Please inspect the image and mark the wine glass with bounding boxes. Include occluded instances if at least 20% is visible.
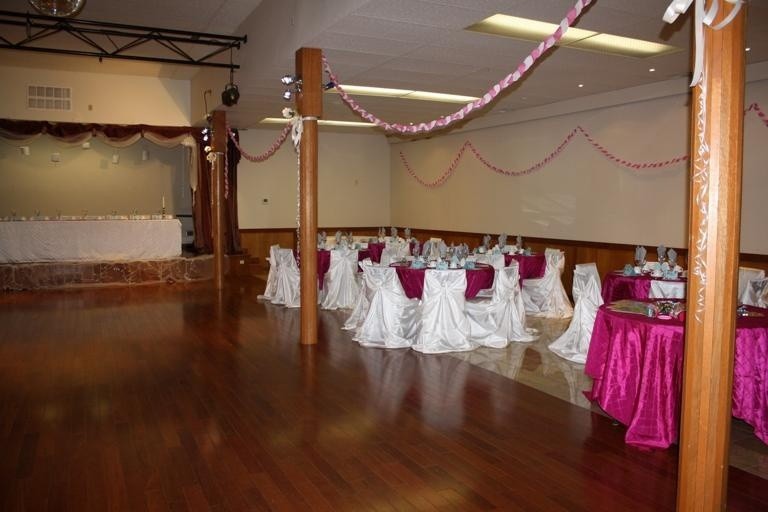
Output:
[413,246,470,268]
[632,255,676,281]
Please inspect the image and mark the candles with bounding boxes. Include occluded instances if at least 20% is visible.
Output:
[162,196,166,208]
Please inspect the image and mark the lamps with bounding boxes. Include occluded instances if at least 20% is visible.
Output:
[201,126,215,144]
[279,71,304,102]
[218,67,242,108]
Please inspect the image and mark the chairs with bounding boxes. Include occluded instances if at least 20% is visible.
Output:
[738,265,767,308]
[545,261,603,364]
[254,225,567,354]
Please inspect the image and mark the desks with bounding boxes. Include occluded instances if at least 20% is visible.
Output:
[0,215,183,264]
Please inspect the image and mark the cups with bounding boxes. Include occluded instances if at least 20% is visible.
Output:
[643,302,686,322]
[479,244,524,254]
